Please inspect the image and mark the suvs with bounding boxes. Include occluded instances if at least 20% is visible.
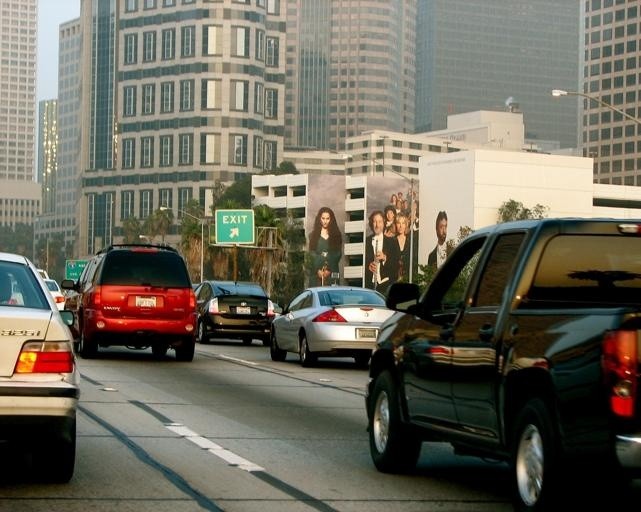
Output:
[61,242,198,362]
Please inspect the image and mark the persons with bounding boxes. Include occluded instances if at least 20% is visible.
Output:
[363,187,419,294]
[308,206,342,286]
[427,211,456,271]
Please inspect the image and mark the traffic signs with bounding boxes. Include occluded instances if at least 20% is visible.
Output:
[65,259,91,281]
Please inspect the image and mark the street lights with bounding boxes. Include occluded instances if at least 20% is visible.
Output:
[337,152,416,282]
[546,86,639,128]
[160,206,207,282]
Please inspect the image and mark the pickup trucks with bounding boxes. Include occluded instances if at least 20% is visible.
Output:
[365,220,640,507]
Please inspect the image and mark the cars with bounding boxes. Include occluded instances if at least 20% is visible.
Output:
[0,252,80,483]
[193,279,277,346]
[268,286,400,368]
